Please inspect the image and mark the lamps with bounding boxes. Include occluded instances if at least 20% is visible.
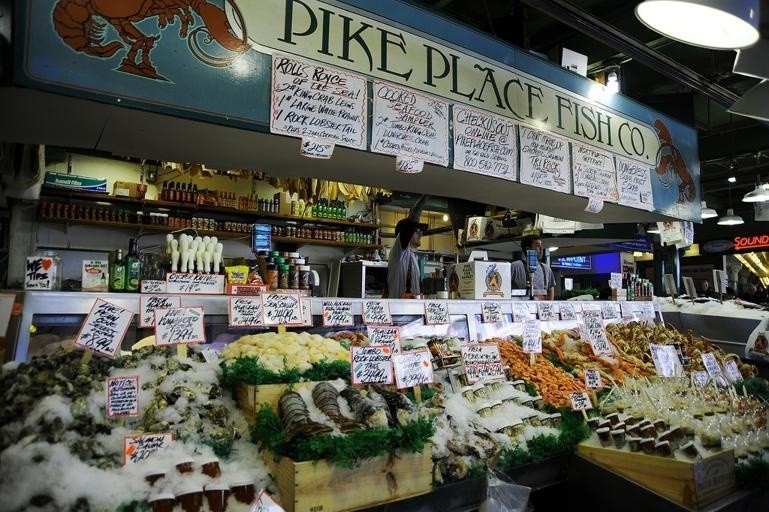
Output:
[603,58,620,94]
[700,23,769,226]
[634,0,761,51]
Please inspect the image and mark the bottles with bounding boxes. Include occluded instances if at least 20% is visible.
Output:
[312,199,346,221]
[162,180,279,212]
[108,235,142,292]
[259,250,311,290]
[272,223,374,244]
[622,273,654,301]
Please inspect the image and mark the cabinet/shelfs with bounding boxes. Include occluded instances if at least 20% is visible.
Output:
[36,145,383,249]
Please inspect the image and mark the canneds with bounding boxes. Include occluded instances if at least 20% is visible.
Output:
[39,199,374,244]
[258,249,311,290]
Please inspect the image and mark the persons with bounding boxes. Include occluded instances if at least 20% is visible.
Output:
[511,234,557,300]
[744,283,769,304]
[386,193,431,299]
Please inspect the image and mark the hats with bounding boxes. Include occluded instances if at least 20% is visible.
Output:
[396,215,429,235]
